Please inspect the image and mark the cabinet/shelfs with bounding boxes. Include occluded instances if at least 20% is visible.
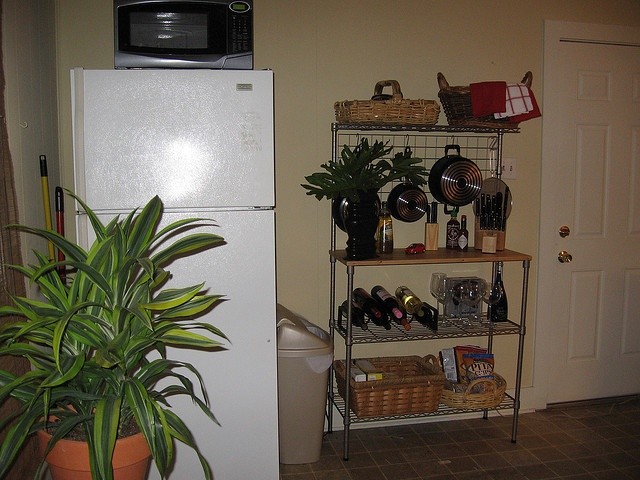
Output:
[323,122,533,461]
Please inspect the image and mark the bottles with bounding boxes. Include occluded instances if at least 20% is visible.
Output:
[447,207,460,250]
[371,285,403,319]
[488,261,508,323]
[378,201,393,254]
[395,285,425,317]
[352,288,381,318]
[415,302,439,330]
[389,296,411,331]
[341,299,368,331]
[371,306,391,330]
[458,216,469,252]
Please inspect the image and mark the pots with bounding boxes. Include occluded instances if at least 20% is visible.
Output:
[429,144,483,215]
[388,146,428,223]
[331,189,381,234]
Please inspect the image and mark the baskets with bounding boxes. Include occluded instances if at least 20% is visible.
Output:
[335,80,440,126]
[436,356,507,409]
[437,72,533,128]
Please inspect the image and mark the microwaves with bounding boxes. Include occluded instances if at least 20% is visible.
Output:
[112,1,255,69]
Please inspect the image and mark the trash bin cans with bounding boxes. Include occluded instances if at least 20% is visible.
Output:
[275,303,333,466]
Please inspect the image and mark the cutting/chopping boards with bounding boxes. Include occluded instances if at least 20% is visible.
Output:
[473,177,513,221]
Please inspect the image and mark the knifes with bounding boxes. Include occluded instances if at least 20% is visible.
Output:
[475,186,509,231]
[425,202,438,223]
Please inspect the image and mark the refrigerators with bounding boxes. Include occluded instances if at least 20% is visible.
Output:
[70,68,280,475]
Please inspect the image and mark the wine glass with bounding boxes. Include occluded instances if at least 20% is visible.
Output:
[452,278,485,331]
[437,278,452,328]
[483,282,502,328]
[429,273,447,317]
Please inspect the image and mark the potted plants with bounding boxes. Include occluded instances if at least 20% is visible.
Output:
[300,137,430,260]
[0,186,234,480]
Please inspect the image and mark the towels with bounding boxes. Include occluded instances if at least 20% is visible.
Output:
[508,88,542,123]
[494,83,534,119]
[469,81,507,117]
